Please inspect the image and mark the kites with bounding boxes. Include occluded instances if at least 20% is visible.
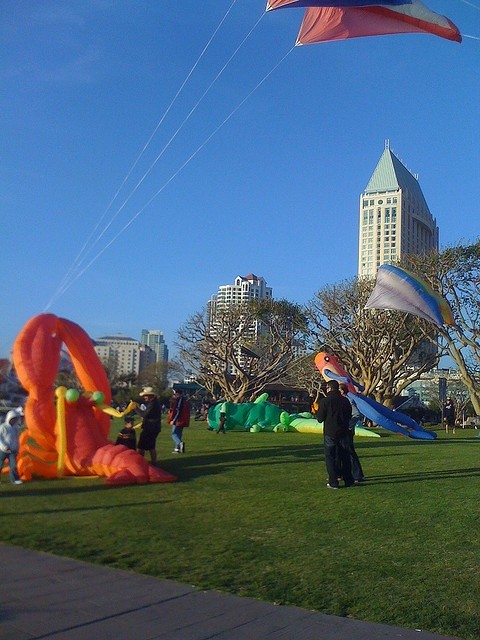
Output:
[262,0,462,49]
[366,262,457,328]
[206,393,382,441]
[314,353,439,442]
[12,314,178,486]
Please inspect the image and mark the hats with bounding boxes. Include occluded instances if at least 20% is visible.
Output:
[124,417,135,423]
[139,386,155,397]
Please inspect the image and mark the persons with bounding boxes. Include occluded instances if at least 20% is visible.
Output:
[167,388,190,453]
[444,396,454,413]
[445,401,456,434]
[337,383,364,486]
[132,387,161,466]
[0,410,23,484]
[315,380,354,488]
[115,417,137,450]
[161,403,165,413]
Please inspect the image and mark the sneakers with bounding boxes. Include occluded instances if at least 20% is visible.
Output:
[327,483,338,490]
[171,448,180,454]
[179,442,185,453]
[11,480,22,485]
[326,476,341,481]
